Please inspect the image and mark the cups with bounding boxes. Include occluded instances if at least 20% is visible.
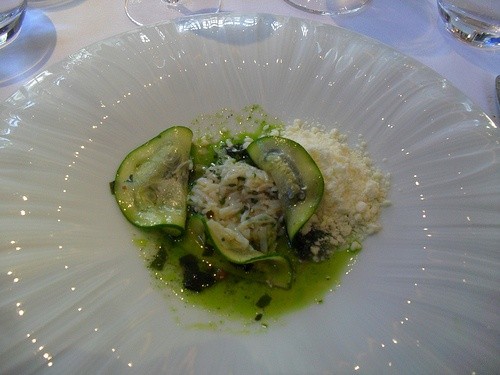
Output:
[436,0,500,49]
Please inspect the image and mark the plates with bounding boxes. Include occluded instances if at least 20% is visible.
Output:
[0,13,500,374]
